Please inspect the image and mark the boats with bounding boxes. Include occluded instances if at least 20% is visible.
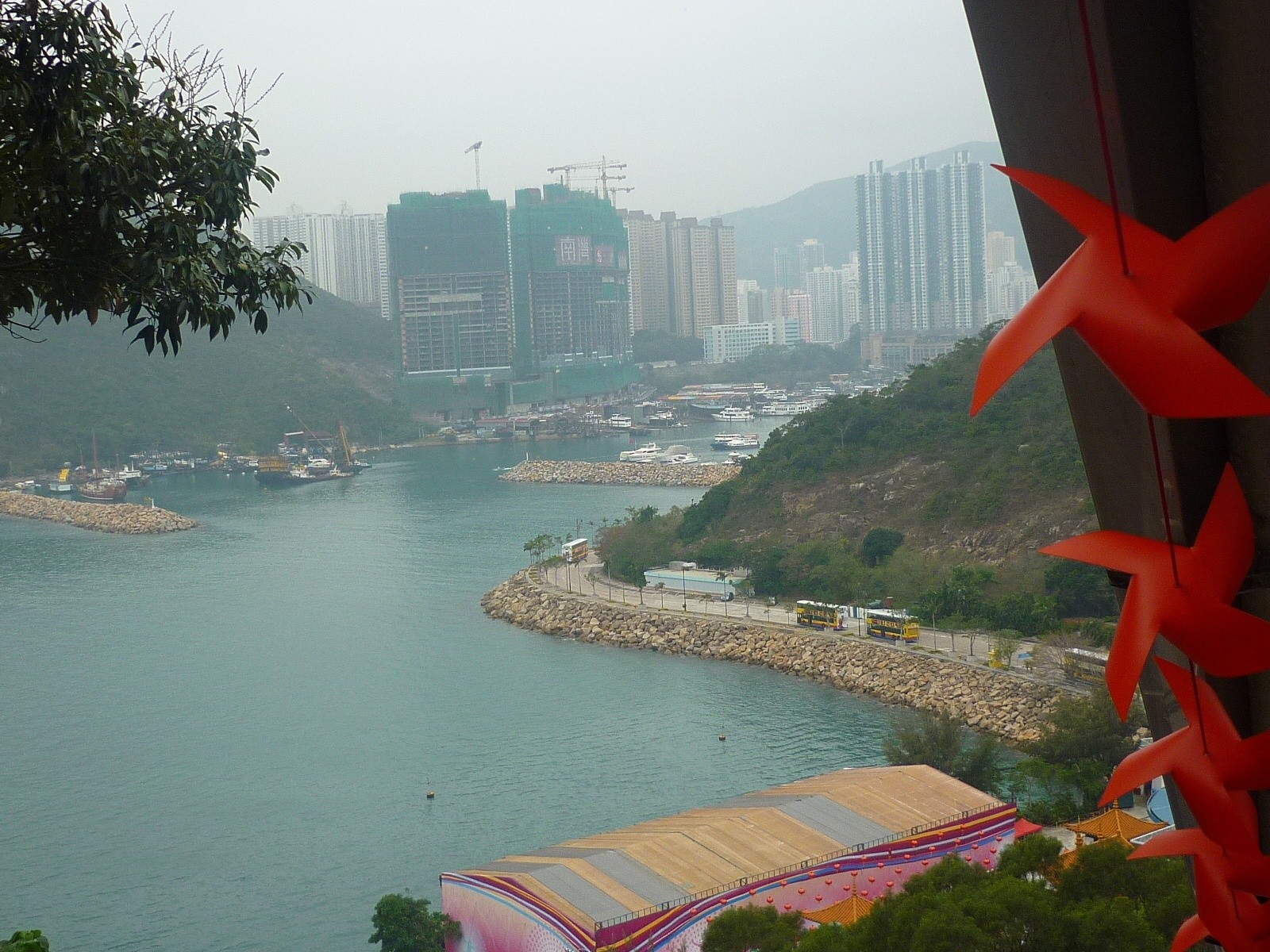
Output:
[710,407,755,422]
[576,411,603,426]
[602,413,632,429]
[756,401,813,416]
[710,432,761,450]
[722,452,753,466]
[15,427,375,505]
[619,441,698,465]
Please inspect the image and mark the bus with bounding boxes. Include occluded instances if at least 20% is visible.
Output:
[1065,649,1140,701]
[866,611,920,643]
[796,600,849,631]
[561,538,589,563]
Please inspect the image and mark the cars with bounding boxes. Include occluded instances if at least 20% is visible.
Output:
[720,592,734,602]
